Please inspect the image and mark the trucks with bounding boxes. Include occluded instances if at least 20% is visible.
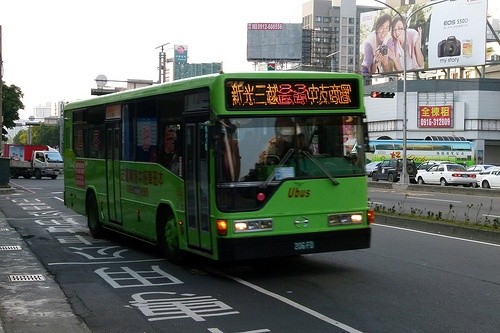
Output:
[10,151,65,180]
[3,144,58,162]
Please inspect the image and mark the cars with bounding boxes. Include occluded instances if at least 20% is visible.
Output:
[363,157,500,191]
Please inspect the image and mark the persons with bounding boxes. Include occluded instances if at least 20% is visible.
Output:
[361,14,395,75]
[387,16,424,71]
[291,133,313,161]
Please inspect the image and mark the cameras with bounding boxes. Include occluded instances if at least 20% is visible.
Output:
[378,45,388,55]
[438,36,461,57]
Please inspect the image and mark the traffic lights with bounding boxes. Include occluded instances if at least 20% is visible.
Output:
[371,90,395,98]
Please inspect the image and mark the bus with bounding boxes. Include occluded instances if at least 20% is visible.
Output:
[63,70,375,266]
